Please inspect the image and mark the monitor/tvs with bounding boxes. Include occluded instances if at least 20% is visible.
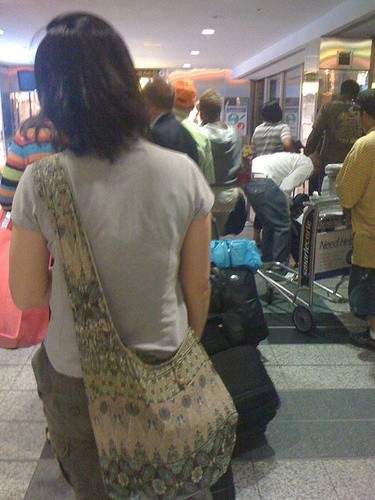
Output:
[17,71,35,91]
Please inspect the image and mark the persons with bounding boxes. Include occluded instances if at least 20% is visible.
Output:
[8,11,214,500]
[335,88,375,348]
[0,80,363,282]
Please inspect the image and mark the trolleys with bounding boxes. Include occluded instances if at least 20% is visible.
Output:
[255,196,351,333]
